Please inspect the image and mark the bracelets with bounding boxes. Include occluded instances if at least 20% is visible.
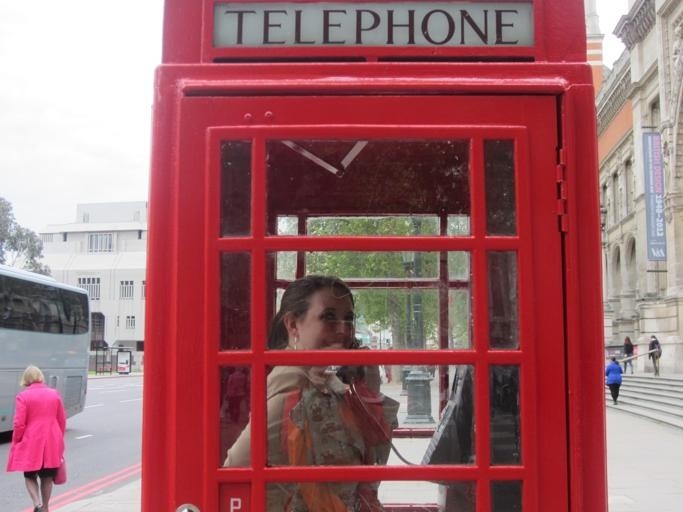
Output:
[359,393,384,404]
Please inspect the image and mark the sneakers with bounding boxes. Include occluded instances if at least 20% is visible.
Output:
[34,505,41,512]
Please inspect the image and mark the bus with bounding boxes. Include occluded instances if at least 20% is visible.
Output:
[0,263,94,433]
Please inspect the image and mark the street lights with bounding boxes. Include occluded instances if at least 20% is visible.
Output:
[396,216,436,429]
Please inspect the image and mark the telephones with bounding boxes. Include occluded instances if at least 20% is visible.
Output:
[335,337,363,385]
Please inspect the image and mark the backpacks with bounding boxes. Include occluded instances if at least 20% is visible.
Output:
[654,344,662,359]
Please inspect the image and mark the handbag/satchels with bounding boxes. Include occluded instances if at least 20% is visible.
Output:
[53,454,67,484]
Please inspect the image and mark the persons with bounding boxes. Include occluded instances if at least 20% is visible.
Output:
[605,356,624,405]
[621,335,635,375]
[226,366,247,426]
[4,364,68,512]
[222,273,401,511]
[647,335,662,375]
